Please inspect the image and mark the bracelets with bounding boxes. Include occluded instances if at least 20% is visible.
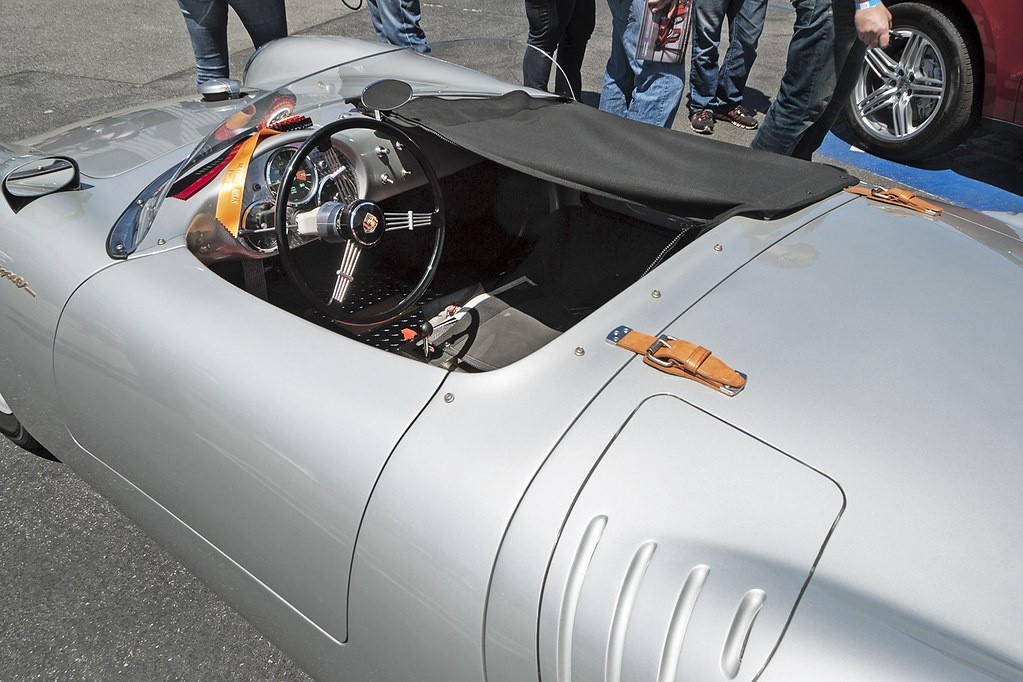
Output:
[854,0,880,10]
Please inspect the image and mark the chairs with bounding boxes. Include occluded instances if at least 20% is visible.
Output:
[391,204,754,372]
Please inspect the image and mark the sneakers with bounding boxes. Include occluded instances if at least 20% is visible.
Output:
[713,105,758,129]
[690,110,713,133]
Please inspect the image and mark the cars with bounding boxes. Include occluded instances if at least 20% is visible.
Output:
[832,0,1022,165]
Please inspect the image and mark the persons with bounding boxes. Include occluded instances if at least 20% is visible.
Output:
[688,0,769,134]
[175,0,290,96]
[599,0,693,131]
[523,0,596,104]
[749,0,893,164]
[365,0,433,57]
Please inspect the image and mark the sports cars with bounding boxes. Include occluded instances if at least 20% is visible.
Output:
[0,32,1023,682]
